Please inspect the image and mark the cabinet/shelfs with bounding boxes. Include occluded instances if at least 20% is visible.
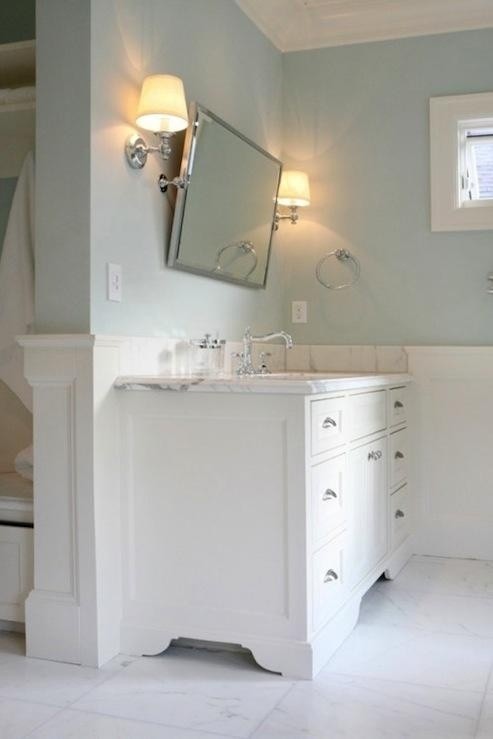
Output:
[113,382,414,680]
[0,496,33,635]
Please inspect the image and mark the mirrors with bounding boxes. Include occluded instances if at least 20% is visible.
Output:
[167,99,284,290]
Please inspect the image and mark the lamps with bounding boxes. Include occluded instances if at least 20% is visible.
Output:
[124,73,190,170]
[273,170,310,231]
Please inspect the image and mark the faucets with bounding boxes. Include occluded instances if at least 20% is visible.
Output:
[243,326,293,375]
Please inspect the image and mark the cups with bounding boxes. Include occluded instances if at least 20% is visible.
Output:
[189,339,226,375]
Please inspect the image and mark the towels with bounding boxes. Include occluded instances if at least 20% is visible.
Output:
[0,148,34,416]
[14,444,33,482]
